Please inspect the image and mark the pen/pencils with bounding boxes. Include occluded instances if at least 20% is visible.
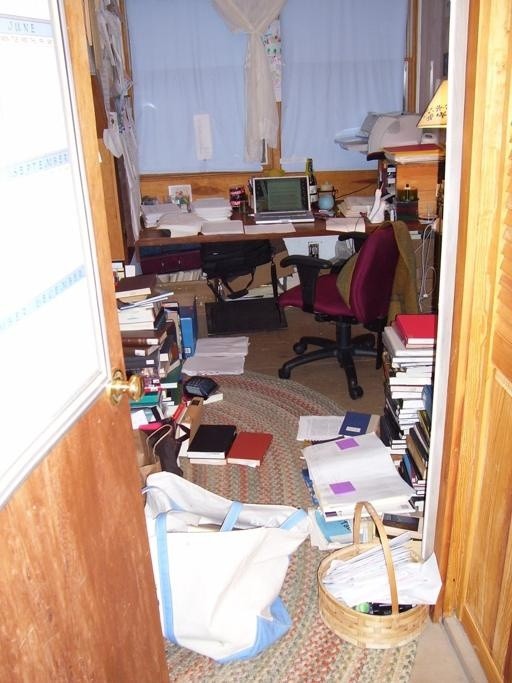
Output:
[247,175,255,195]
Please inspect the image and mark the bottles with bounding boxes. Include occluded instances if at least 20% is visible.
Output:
[318,180,334,211]
[303,157,319,208]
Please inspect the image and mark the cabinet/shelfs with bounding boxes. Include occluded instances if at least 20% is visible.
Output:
[378,159,440,219]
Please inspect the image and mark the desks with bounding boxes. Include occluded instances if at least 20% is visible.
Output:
[135,211,340,260]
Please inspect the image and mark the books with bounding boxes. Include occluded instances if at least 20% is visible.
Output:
[338,143,445,222]
[112,261,274,469]
[296,313,439,551]
[139,197,296,234]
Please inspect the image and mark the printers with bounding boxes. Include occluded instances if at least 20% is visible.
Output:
[334,110,425,153]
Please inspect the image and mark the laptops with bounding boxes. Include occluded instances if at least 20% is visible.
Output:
[253,175,316,224]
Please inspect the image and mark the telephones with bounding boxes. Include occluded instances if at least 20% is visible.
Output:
[366,181,387,224]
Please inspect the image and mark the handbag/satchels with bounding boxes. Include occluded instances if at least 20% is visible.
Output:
[147,418,183,477]
[139,471,314,662]
[201,239,272,279]
[133,431,162,479]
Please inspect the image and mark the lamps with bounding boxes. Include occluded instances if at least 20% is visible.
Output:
[416,79,447,129]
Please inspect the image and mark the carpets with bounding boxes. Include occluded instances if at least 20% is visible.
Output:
[163,370,417,683]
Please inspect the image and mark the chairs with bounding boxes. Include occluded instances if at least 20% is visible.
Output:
[277,225,399,400]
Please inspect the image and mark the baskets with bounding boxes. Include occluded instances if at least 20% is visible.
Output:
[317,502,431,650]
